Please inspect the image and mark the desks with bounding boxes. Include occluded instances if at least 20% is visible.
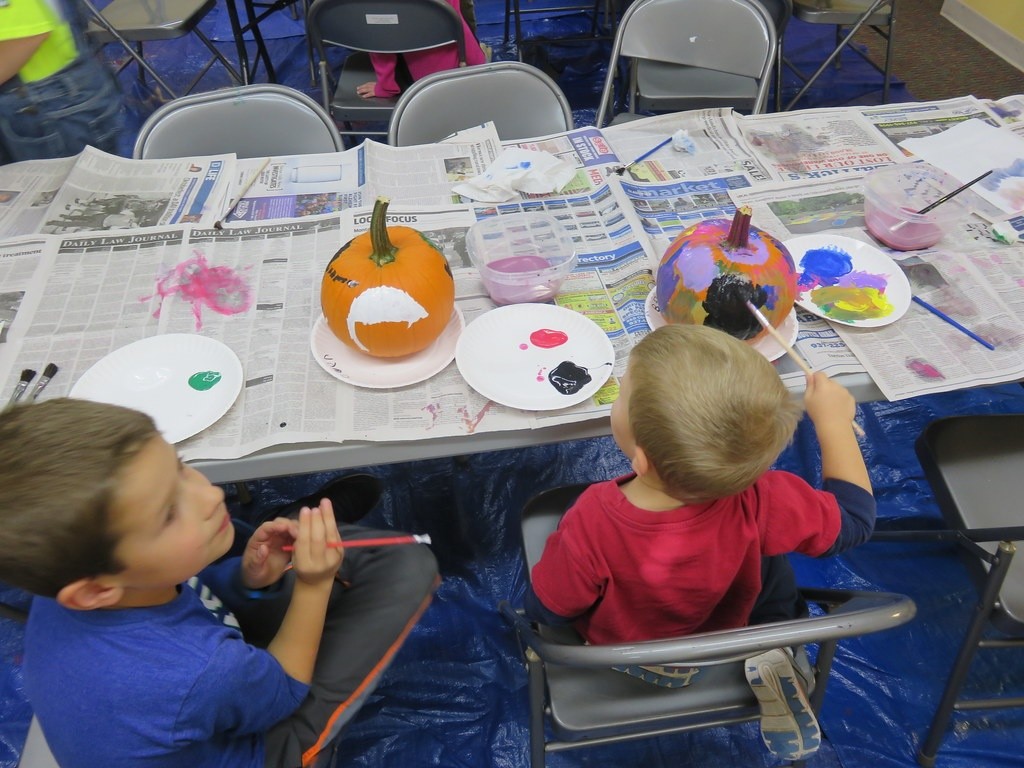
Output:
[1,94,1024,486]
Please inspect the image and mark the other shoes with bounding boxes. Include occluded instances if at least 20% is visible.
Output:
[608,656,704,688]
[745,649,823,760]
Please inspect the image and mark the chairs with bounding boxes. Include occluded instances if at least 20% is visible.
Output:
[498,479,917,768]
[864,414,1024,768]
[80,0,895,160]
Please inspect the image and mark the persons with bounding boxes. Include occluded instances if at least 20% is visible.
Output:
[0,398,443,768]
[0,0,136,160]
[525,324,874,761]
[356,0,493,98]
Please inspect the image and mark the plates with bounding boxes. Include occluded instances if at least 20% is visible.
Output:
[309,302,466,389]
[456,303,615,410]
[781,235,912,327]
[645,285,798,362]
[68,333,243,445]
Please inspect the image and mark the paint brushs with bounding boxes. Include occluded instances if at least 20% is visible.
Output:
[213,158,272,230]
[746,299,866,437]
[282,533,431,552]
[616,137,673,176]
[9,369,36,404]
[911,294,995,350]
[890,169,993,231]
[24,362,58,403]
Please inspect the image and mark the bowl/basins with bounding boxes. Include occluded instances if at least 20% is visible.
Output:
[466,211,578,307]
[864,163,977,250]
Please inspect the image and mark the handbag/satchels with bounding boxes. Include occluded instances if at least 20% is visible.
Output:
[520,29,629,108]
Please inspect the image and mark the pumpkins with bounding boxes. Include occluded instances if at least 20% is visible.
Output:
[656,205,796,346]
[321,195,455,359]
